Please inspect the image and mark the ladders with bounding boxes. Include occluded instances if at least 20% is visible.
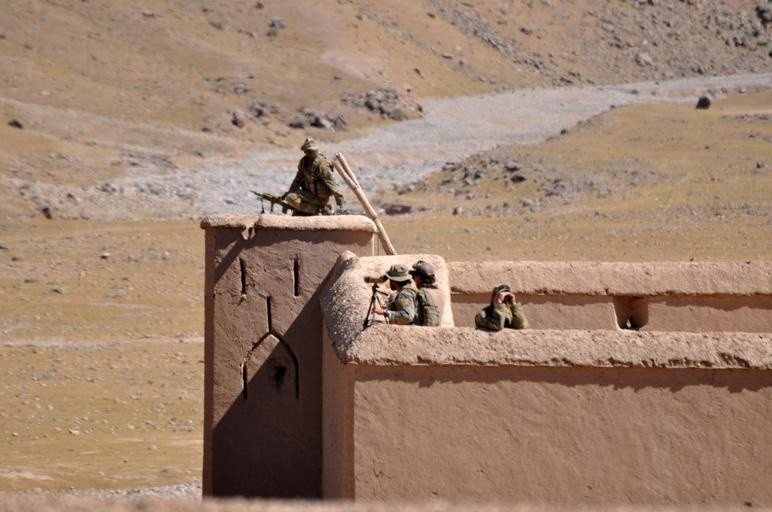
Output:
[334,152,397,255]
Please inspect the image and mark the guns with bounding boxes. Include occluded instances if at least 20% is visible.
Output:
[250,191,312,215]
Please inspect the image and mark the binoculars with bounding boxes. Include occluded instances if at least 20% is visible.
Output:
[505,294,513,300]
[364,282,390,325]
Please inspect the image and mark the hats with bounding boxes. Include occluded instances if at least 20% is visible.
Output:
[408,260,435,280]
[384,262,412,282]
[491,283,511,296]
[301,137,319,152]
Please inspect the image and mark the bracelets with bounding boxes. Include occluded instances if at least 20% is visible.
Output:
[383,309,388,317]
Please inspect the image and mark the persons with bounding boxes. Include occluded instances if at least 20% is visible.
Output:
[372,263,419,325]
[409,260,444,326]
[474,284,529,331]
[286,138,346,214]
